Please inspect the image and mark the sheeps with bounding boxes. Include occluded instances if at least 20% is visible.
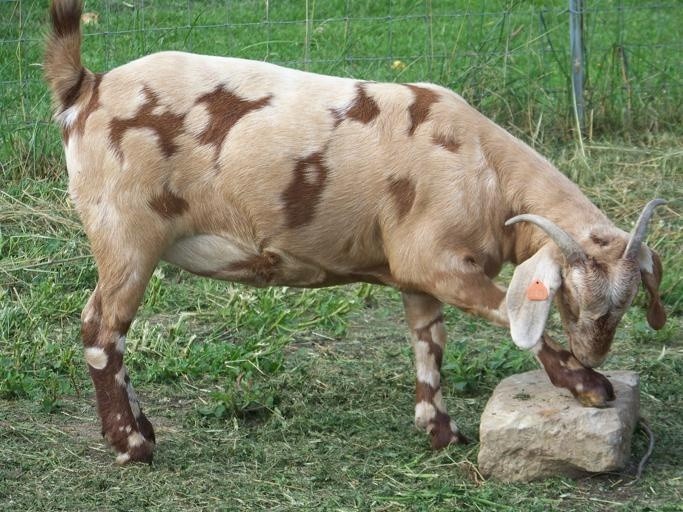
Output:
[41,0,667,468]
